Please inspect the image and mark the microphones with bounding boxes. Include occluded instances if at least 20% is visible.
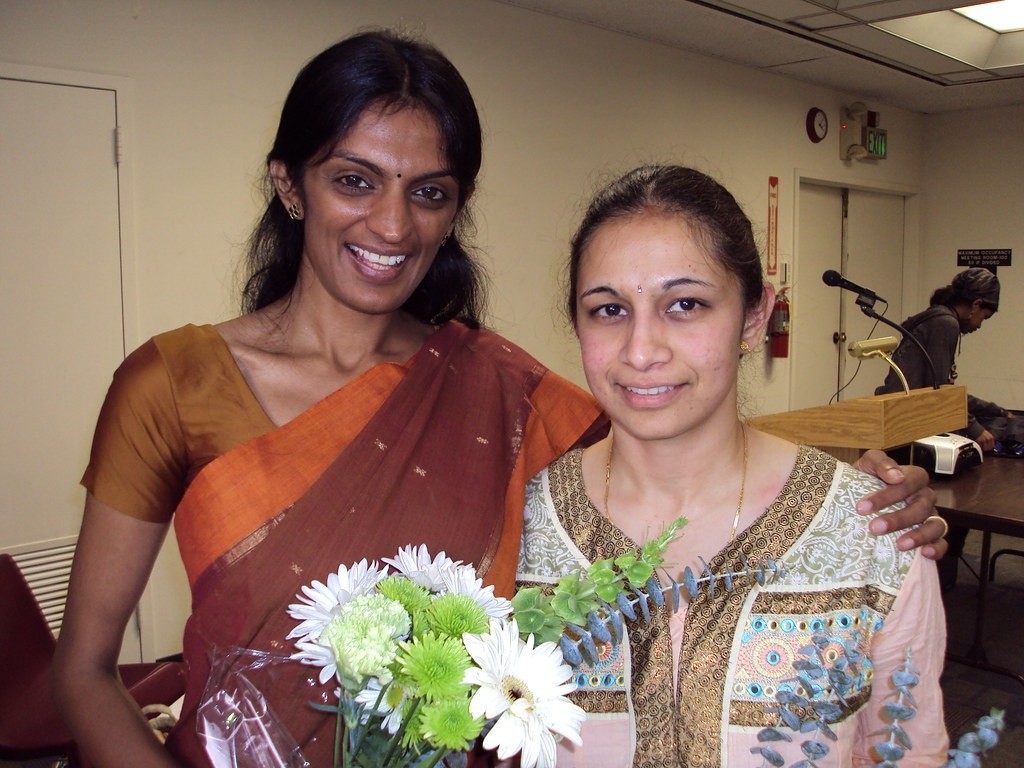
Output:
[822,270,888,306]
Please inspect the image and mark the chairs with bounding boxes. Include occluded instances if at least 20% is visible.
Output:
[0,553,187,768]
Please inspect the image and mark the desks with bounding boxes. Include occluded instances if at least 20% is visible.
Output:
[928,456,1024,687]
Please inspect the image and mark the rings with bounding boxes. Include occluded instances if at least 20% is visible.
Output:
[924,515,948,538]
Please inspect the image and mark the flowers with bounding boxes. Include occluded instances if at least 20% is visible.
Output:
[750,618,1008,768]
[283,517,788,768]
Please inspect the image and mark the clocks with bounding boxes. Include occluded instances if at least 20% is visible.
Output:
[806,107,829,144]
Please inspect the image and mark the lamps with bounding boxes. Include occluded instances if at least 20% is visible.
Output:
[838,101,867,161]
[848,336,911,395]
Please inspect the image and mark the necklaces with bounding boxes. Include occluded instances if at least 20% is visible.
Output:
[604,418,749,545]
[951,305,960,321]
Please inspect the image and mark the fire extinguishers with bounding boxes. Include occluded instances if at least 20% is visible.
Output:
[764,287,790,357]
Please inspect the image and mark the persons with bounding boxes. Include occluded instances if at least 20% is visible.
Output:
[49,24,951,768]
[884,267,1018,596]
[512,164,949,768]
